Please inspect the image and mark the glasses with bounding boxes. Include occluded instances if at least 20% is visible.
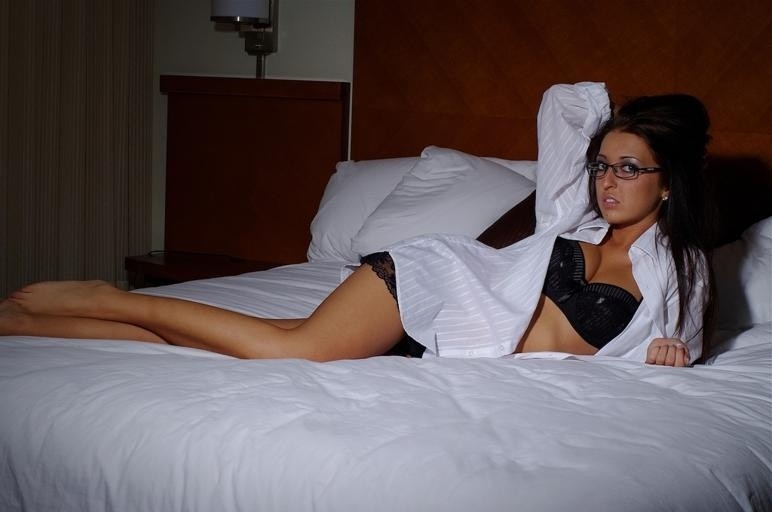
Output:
[586,161,660,179]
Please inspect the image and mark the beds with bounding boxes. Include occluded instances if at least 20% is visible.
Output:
[1,261,771,511]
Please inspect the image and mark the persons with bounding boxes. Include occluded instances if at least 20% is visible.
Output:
[0,80,712,368]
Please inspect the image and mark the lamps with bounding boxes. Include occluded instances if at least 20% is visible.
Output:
[209,1,279,79]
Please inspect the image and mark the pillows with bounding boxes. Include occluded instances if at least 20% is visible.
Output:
[340,148,531,285]
[703,222,768,337]
[477,190,536,248]
[305,157,535,263]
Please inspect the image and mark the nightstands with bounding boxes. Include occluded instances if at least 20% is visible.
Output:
[125,254,285,294]
